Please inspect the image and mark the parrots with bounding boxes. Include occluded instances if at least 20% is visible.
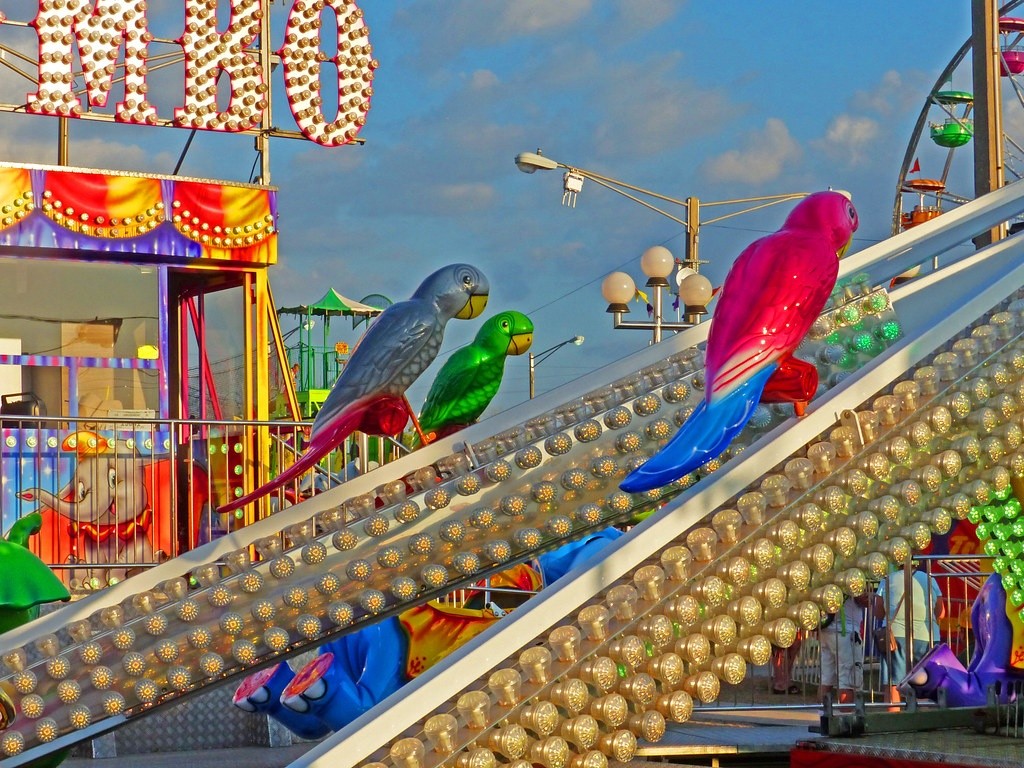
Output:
[616,190,858,493]
[218,264,489,514]
[413,312,534,452]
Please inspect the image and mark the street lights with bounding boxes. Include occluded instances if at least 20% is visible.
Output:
[528,333,586,400]
[512,148,853,289]
[268,317,314,404]
[604,244,711,346]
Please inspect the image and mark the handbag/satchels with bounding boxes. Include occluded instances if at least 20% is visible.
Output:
[873,626,897,653]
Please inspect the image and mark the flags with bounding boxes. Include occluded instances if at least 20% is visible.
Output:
[635,288,653,318]
[672,297,679,310]
[909,158,920,173]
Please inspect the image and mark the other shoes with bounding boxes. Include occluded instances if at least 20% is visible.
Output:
[773,685,801,695]
[817,709,824,716]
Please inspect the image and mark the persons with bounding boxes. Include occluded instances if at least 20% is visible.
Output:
[291,364,299,382]
[772,629,801,693]
[874,564,946,711]
[819,584,870,715]
